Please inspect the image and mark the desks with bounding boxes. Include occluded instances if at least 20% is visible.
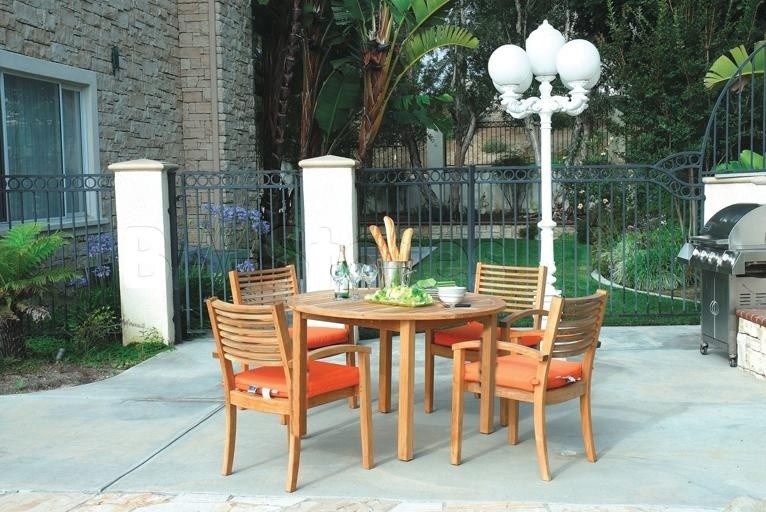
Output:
[285,287,507,461]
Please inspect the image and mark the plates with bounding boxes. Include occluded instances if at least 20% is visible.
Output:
[424,288,439,297]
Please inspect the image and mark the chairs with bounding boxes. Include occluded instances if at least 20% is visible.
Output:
[205,263,371,491]
[425,260,548,427]
[451,288,608,481]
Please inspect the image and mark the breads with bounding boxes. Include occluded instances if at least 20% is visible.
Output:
[399,228,414,261]
[383,216,399,261]
[369,225,392,262]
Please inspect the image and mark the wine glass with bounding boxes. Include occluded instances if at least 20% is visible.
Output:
[359,263,379,299]
[328,262,346,300]
[348,263,361,301]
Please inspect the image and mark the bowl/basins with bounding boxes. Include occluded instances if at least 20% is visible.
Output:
[437,286,468,306]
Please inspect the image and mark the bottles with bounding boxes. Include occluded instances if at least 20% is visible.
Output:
[333,244,349,300]
